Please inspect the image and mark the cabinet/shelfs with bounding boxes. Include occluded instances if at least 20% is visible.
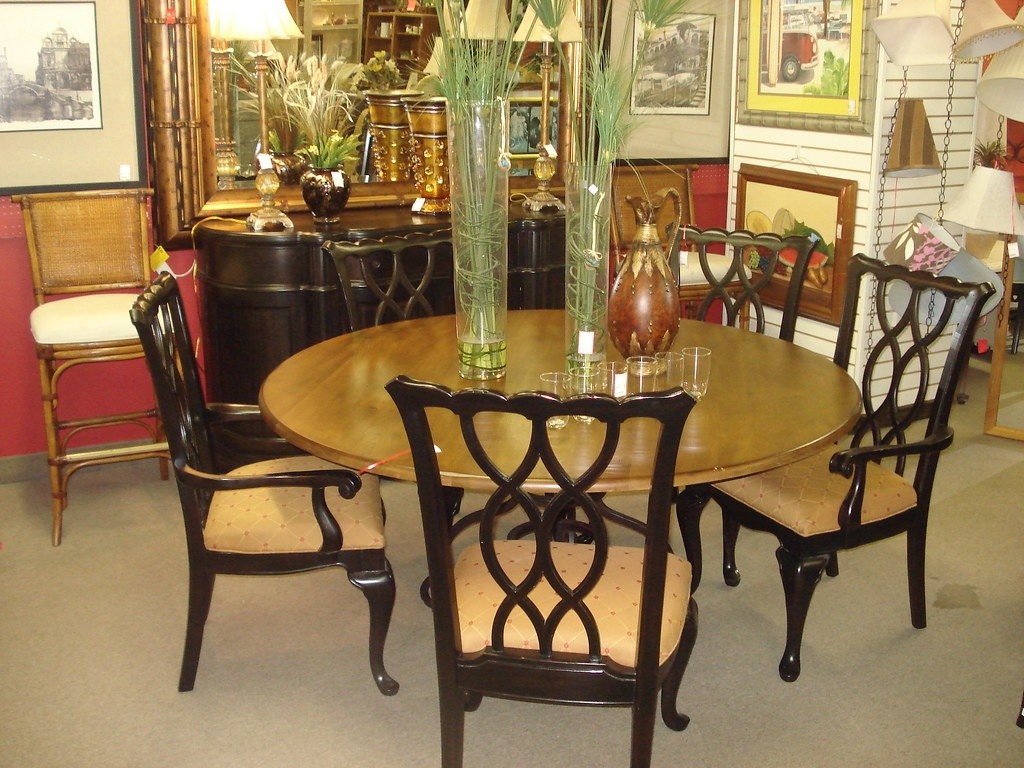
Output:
[271,0,440,82]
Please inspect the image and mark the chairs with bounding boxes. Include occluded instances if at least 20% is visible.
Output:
[128,235,995,768]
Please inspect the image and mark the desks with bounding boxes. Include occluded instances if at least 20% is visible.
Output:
[191,192,565,473]
[257,309,864,598]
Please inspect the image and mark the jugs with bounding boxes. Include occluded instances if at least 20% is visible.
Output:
[607,185,683,374]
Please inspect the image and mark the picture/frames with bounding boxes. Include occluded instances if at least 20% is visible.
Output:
[629,9,716,116]
[734,163,858,327]
[0,2,102,132]
[734,0,882,137]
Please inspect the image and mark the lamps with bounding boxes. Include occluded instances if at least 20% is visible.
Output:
[206,1,291,192]
[455,0,516,55]
[207,0,306,235]
[511,0,587,213]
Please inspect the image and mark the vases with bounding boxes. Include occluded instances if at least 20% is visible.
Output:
[272,152,302,186]
[562,162,615,390]
[214,65,241,177]
[299,164,352,223]
[443,101,512,381]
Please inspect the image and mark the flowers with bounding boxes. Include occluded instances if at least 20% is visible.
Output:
[269,53,362,167]
[225,43,406,152]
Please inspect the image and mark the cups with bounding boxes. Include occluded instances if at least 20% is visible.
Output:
[568,366,601,423]
[539,372,574,430]
[654,351,685,392]
[625,356,658,396]
[597,361,629,400]
[681,347,712,399]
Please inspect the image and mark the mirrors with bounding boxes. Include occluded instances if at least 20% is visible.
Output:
[985,193,1024,443]
[135,0,603,250]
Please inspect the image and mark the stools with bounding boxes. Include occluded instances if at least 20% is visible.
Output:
[611,165,751,332]
[10,188,183,547]
